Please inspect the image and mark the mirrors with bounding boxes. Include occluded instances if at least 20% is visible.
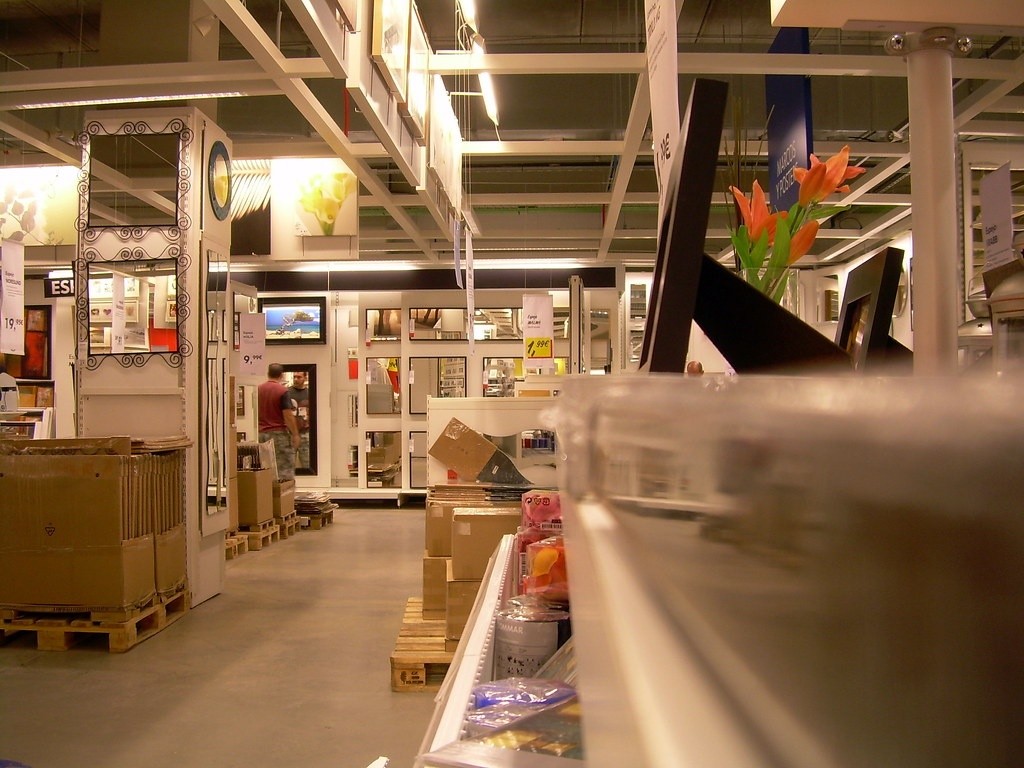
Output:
[74,118,195,244]
[278,361,317,476]
[71,245,193,370]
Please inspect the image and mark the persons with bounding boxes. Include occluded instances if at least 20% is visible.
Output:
[258,363,300,480]
[286,372,310,468]
[687,361,704,377]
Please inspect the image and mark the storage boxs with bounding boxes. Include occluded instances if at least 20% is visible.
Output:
[272,478,297,516]
[419,487,530,650]
[236,467,276,525]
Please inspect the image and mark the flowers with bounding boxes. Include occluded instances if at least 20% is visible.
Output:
[718,82,870,304]
